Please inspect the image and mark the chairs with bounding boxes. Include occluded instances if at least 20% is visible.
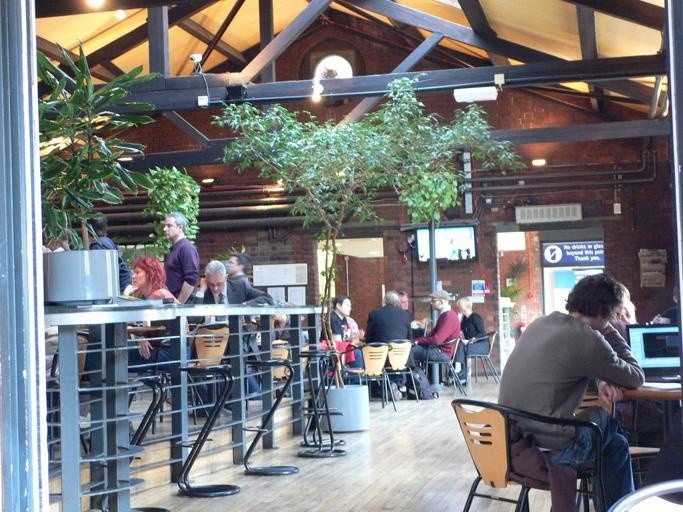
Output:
[42,327,90,459]
[339,329,501,412]
[447,397,609,512]
[155,323,228,425]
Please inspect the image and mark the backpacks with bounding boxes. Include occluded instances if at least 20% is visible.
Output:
[405,366,439,400]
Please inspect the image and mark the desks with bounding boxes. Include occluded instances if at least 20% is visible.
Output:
[579,381,682,494]
[41,297,329,512]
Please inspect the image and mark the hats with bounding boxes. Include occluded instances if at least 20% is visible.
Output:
[427,290,448,300]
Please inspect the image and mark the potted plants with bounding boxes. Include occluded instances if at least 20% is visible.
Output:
[208,71,440,435]
[500,282,521,306]
[34,43,162,307]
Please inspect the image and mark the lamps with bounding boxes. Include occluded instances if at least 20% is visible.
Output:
[491,72,507,94]
[611,186,622,214]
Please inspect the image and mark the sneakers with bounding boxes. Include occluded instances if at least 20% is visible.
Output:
[390,381,403,401]
[452,382,466,386]
[450,371,464,378]
[397,381,407,393]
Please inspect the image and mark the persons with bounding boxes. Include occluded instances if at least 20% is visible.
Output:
[43,210,287,416]
[320,287,489,403]
[614,282,641,345]
[495,274,645,506]
[651,286,680,326]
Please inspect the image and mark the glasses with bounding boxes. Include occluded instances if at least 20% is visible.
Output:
[432,300,440,303]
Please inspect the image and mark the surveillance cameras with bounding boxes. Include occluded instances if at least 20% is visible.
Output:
[189,53,202,62]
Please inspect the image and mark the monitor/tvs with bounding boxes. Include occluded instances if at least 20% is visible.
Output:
[416,225,479,264]
[626,324,683,383]
